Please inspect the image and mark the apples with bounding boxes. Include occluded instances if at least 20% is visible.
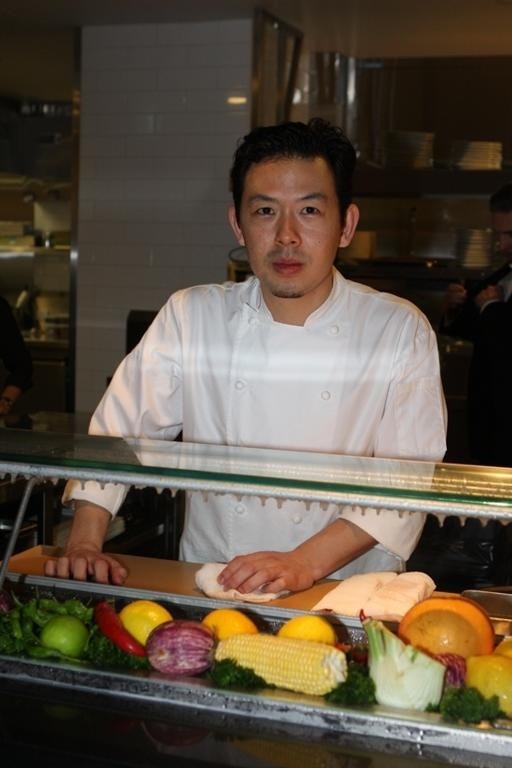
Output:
[39,614,89,657]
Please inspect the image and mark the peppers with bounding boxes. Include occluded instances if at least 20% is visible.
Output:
[0,586,93,649]
[95,599,148,657]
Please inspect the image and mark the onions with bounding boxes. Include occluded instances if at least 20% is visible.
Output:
[145,620,215,676]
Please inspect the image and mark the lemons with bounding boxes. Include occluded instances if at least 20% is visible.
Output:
[466,654,512,719]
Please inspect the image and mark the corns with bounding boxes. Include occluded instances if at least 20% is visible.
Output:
[215,633,348,695]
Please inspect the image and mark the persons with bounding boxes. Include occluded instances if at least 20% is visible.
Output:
[436,180,510,467]
[429,261,483,340]
[0,293,34,428]
[45,117,448,597]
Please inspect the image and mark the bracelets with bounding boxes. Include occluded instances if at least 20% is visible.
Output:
[0,396,14,409]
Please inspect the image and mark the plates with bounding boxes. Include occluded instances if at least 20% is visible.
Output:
[376,128,504,172]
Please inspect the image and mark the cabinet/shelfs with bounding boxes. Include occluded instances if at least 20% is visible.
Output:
[0,429,512,768]
[334,164,512,280]
[0,92,79,411]
